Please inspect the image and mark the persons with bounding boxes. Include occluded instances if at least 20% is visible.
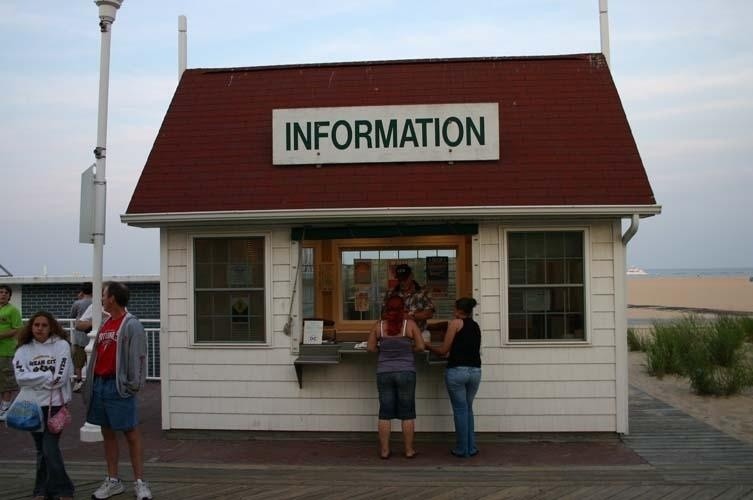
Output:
[77,288,83,299]
[0,284,23,422]
[76,301,109,334]
[366,296,425,459]
[69,281,93,391]
[11,309,78,500]
[423,296,483,457]
[80,281,153,500]
[391,263,436,332]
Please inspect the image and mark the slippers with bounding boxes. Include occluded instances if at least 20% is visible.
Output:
[378,449,419,458]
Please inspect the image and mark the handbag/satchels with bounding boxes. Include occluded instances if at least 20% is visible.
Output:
[46,405,71,435]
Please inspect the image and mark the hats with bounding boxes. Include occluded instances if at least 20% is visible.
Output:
[453,296,476,313]
[394,264,411,279]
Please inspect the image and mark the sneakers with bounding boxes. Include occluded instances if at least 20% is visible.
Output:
[72,379,83,393]
[451,448,478,458]
[0,399,10,410]
[133,478,152,500]
[90,477,124,499]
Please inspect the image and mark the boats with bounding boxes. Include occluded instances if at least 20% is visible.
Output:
[624,265,648,276]
